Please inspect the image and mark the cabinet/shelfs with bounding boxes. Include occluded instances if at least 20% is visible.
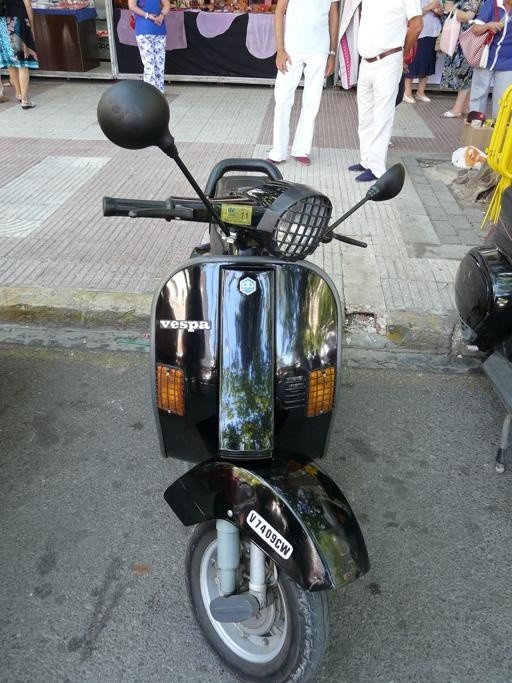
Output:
[28,4,103,73]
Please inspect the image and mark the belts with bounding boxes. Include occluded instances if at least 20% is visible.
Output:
[362,46,404,63]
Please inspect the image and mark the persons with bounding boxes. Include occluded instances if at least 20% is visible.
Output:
[268,0,342,164]
[0,0,38,110]
[437,0,498,118]
[402,0,443,104]
[347,0,425,183]
[463,0,512,127]
[0,78,10,104]
[127,0,171,94]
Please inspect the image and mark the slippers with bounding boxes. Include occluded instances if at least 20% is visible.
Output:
[439,111,461,118]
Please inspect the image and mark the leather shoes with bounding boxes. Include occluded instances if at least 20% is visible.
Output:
[266,156,311,166]
[0,94,36,110]
[347,163,378,182]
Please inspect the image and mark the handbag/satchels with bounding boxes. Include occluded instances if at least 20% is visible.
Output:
[440,19,461,57]
[459,23,495,69]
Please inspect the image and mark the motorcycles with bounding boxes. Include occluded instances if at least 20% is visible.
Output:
[98,80,404,683]
[449,145,512,472]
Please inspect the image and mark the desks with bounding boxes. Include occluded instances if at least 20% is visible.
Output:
[109,5,281,77]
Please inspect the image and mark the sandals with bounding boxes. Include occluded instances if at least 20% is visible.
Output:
[403,92,431,103]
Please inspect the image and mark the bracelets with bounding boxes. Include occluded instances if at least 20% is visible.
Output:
[161,11,166,15]
[328,50,337,56]
[145,12,149,20]
